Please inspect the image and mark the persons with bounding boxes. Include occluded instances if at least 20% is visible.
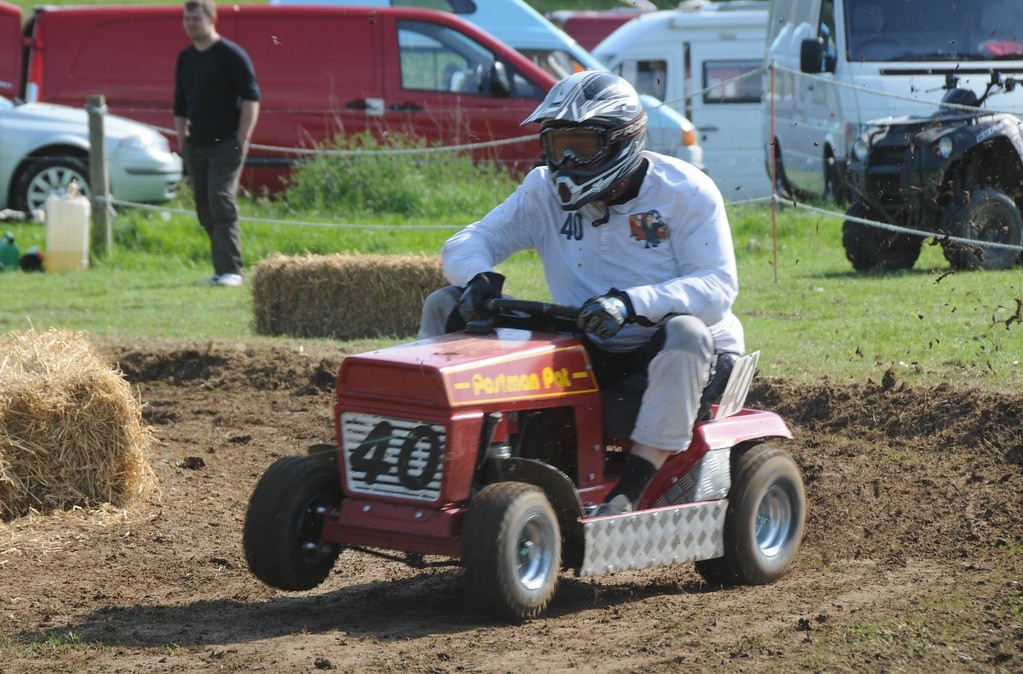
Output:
[172,0,261,286]
[417,71,744,509]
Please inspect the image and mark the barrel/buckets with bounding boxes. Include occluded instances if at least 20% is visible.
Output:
[44,181,90,274]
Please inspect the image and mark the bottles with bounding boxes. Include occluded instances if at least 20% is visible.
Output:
[0,238,19,274]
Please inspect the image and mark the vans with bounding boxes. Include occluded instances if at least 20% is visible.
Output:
[274,0,705,171]
[0,0,564,202]
[761,0,1023,211]
[586,0,770,201]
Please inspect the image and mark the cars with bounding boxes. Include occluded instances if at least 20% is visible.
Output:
[0,93,185,220]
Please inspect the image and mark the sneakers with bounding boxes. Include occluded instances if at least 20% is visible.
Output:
[586,494,632,518]
[216,272,242,287]
[198,275,222,285]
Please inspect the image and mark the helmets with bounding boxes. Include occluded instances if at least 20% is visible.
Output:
[518,70,648,211]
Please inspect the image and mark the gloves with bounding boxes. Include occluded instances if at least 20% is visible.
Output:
[578,288,634,340]
[459,272,505,321]
